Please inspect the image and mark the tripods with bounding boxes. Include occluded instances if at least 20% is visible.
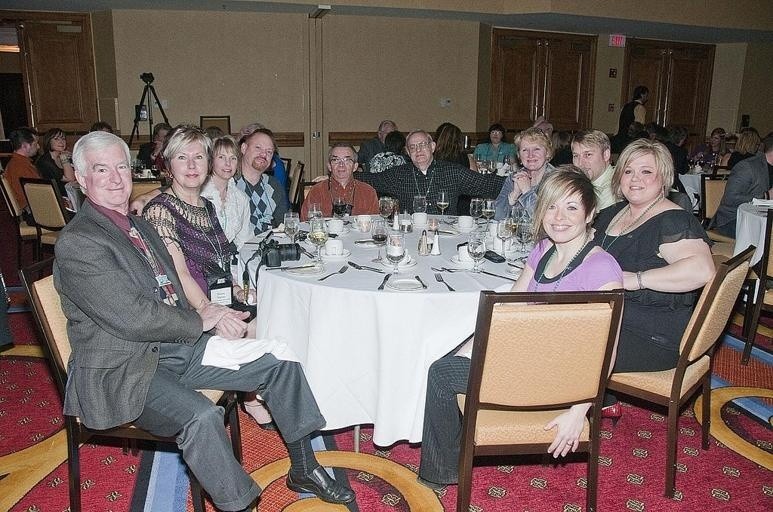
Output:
[127,71,173,149]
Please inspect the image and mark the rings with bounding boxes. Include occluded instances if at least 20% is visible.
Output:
[566,442,573,447]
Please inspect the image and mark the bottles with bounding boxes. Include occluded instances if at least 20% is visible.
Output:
[430,229,440,256]
[419,230,429,255]
[392,210,400,231]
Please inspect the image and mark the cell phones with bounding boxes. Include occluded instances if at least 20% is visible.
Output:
[484,248,506,265]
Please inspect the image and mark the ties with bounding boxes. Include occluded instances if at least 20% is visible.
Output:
[129,224,180,307]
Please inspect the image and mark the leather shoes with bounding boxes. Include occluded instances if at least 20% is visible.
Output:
[286,465,356,505]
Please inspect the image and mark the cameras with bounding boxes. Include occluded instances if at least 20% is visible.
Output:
[253,229,305,270]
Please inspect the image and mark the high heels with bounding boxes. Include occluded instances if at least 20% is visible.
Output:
[240,401,279,431]
[600,403,622,426]
[417,476,448,491]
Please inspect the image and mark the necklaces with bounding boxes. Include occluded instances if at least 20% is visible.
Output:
[172,185,226,273]
[600,195,663,252]
[534,234,588,292]
[412,160,435,206]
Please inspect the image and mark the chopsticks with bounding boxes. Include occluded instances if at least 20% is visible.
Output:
[245,242,260,245]
[480,271,517,282]
[478,221,494,226]
[266,265,315,270]
[505,262,524,270]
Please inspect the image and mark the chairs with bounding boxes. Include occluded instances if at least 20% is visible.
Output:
[709,165,731,180]
[288,162,306,204]
[606,245,756,497]
[740,209,773,365]
[1,175,55,271]
[19,175,71,280]
[457,289,623,512]
[19,255,242,512]
[300,180,318,208]
[700,174,737,244]
[279,158,292,178]
[0,152,15,171]
[712,253,758,337]
[129,177,166,204]
[199,115,231,138]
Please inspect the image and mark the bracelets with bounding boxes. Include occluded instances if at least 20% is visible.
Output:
[636,270,645,290]
[766,190,770,200]
[60,157,70,164]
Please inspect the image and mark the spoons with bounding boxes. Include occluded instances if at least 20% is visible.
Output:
[348,262,385,272]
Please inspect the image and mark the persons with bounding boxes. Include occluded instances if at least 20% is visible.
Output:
[37,127,76,197]
[417,165,625,493]
[5,127,45,226]
[703,127,732,162]
[494,127,558,222]
[240,122,290,212]
[232,130,287,235]
[655,127,672,144]
[534,116,554,139]
[720,127,761,170]
[708,131,773,241]
[300,141,380,222]
[137,122,173,176]
[87,120,114,134]
[30,127,43,167]
[619,85,650,135]
[645,121,659,140]
[473,124,516,165]
[432,122,471,169]
[658,124,692,212]
[202,125,224,141]
[200,134,250,284]
[52,129,356,512]
[571,128,625,215]
[312,129,507,216]
[628,121,644,140]
[140,123,280,432]
[587,139,716,425]
[366,131,412,174]
[357,120,399,172]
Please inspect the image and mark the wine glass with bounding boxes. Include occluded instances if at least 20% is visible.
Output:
[429,217,440,233]
[379,197,394,219]
[285,212,301,244]
[468,235,486,274]
[507,207,519,232]
[151,164,162,184]
[310,219,328,262]
[333,197,348,219]
[413,195,427,212]
[470,198,485,223]
[387,234,405,279]
[372,220,388,263]
[308,203,322,222]
[497,220,512,260]
[483,200,497,232]
[516,223,533,255]
[437,191,449,215]
[489,160,496,173]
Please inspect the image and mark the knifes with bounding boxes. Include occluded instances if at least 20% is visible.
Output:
[413,275,427,289]
[378,273,392,291]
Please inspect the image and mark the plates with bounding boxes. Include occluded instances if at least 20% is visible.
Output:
[380,257,418,268]
[451,255,486,266]
[323,249,351,259]
[286,263,326,276]
[452,224,476,233]
[459,246,473,262]
[385,279,428,290]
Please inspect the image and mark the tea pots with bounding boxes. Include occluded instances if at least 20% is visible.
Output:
[695,165,701,174]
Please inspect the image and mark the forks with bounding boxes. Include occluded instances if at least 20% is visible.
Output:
[319,265,350,281]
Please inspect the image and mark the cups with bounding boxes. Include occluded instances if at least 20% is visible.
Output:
[485,231,502,251]
[398,214,412,234]
[396,248,411,265]
[489,224,498,237]
[355,215,371,234]
[412,213,428,227]
[458,216,474,230]
[506,265,525,278]
[326,220,343,236]
[325,240,344,256]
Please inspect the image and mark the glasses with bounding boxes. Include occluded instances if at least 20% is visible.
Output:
[490,131,502,135]
[409,141,430,152]
[329,157,355,164]
